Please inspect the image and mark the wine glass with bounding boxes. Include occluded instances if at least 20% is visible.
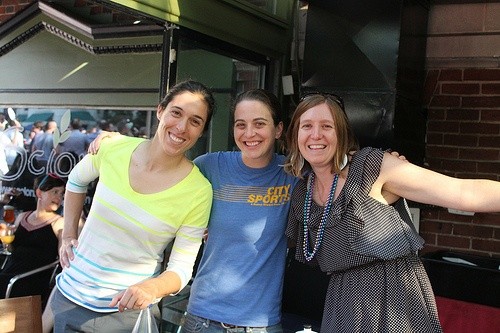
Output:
[0,223,14,255]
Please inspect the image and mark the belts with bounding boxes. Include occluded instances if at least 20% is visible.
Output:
[220,322,235,329]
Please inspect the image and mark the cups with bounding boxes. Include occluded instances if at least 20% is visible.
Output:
[3,206,15,226]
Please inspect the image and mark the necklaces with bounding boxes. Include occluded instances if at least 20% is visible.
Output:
[300,166,339,262]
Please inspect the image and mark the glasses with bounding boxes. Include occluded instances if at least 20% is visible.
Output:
[297,91,345,112]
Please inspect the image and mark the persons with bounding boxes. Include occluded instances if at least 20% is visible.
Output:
[285,91,499,333]
[89,91,408,333]
[0,106,151,216]
[1,173,87,297]
[43,81,214,333]
